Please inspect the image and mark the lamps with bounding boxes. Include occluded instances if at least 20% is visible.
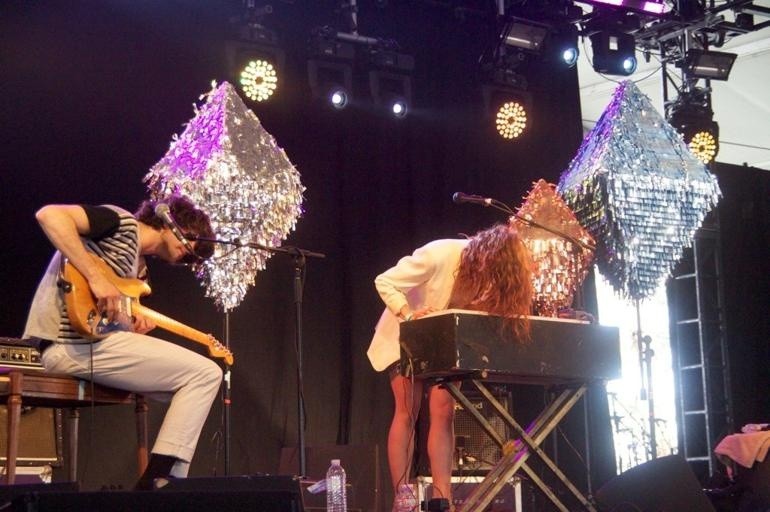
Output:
[229,36,285,113]
[481,85,536,147]
[667,88,721,165]
[546,25,640,77]
[669,45,739,82]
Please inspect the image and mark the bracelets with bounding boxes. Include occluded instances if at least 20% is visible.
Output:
[405,311,415,320]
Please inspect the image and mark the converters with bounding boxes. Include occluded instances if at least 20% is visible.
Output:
[421,498,450,512]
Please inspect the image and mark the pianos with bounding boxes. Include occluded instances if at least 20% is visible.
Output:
[399,308,620,387]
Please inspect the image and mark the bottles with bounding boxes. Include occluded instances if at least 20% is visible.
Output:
[326,459,347,512]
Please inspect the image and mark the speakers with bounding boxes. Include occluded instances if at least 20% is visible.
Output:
[418,391,513,476]
[592,456,716,512]
[155,476,304,512]
[0,365,63,467]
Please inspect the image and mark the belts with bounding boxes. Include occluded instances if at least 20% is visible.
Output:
[39,340,52,354]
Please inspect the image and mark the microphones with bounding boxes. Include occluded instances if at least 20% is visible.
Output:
[452,191,492,206]
[454,436,466,465]
[154,203,193,253]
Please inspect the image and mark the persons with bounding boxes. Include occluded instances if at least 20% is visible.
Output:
[366,222,534,512]
[21,194,226,491]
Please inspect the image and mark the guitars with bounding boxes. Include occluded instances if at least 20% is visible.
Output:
[57,252,235,367]
